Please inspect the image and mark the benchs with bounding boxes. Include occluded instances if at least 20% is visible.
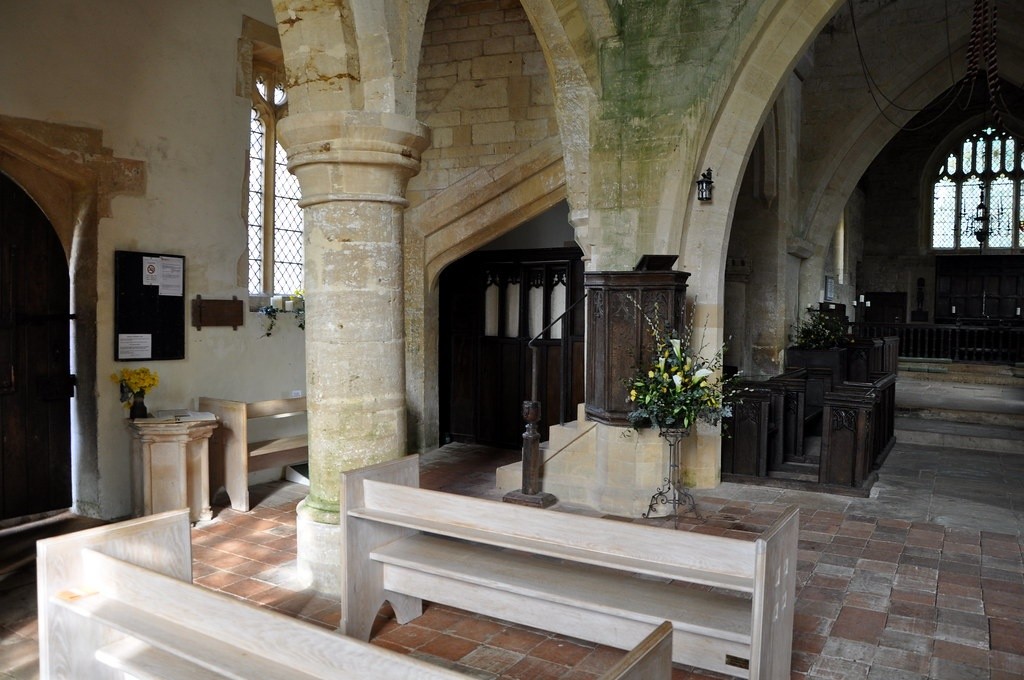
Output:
[34,506,674,679]
[336,453,801,679]
[199,395,308,514]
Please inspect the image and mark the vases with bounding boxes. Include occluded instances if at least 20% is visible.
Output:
[129,390,148,419]
[659,426,688,435]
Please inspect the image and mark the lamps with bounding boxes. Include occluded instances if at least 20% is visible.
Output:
[696,168,714,201]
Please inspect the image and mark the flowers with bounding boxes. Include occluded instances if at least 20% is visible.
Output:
[111,367,158,410]
[789,306,855,351]
[621,298,756,442]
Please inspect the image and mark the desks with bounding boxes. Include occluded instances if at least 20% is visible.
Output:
[124,410,219,526]
